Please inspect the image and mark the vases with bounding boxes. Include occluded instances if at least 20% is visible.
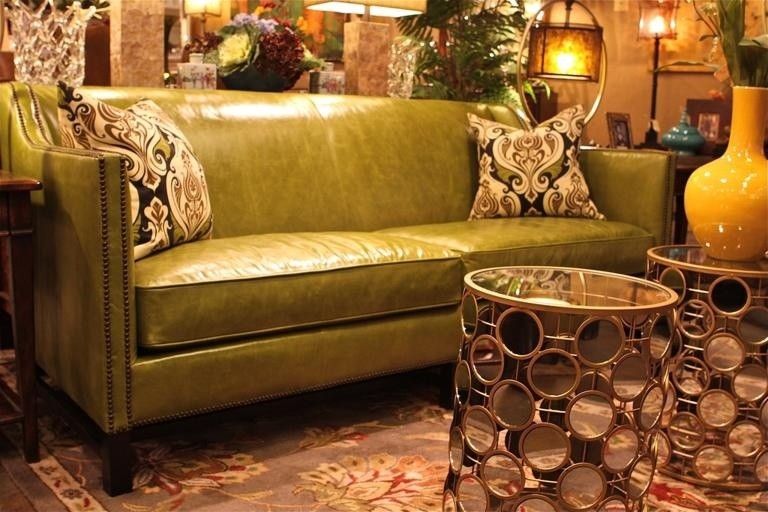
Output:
[683,85,768,261]
[219,61,304,92]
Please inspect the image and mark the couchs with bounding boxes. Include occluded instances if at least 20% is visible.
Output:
[0,80,678,497]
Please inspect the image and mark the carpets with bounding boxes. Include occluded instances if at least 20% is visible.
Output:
[0,335,768,512]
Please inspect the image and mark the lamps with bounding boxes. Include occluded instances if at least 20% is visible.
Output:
[182,0,222,63]
[527,0,604,83]
[634,0,680,151]
[303,0,428,96]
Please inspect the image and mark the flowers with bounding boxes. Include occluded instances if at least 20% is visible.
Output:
[648,0,768,86]
[205,0,327,80]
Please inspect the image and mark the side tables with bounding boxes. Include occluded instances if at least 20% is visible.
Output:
[0,166,44,464]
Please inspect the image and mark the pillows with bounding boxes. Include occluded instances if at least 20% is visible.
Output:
[56,80,214,262]
[465,103,606,223]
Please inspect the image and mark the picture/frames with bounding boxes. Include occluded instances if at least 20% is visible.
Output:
[685,98,733,156]
[606,112,634,150]
